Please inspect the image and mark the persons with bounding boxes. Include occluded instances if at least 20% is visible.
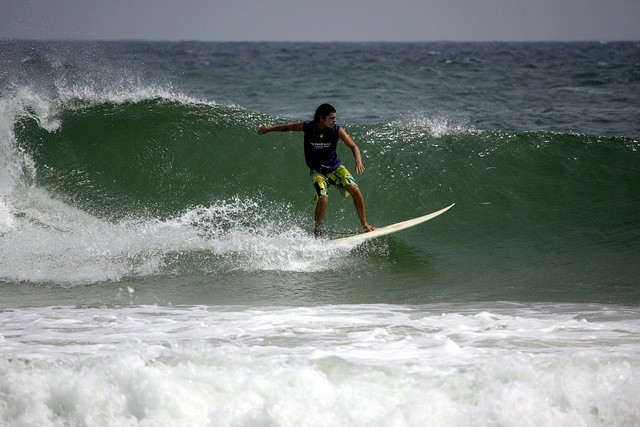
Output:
[257,103,378,238]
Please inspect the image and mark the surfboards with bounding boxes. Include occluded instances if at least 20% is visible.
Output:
[328,202,456,247]
